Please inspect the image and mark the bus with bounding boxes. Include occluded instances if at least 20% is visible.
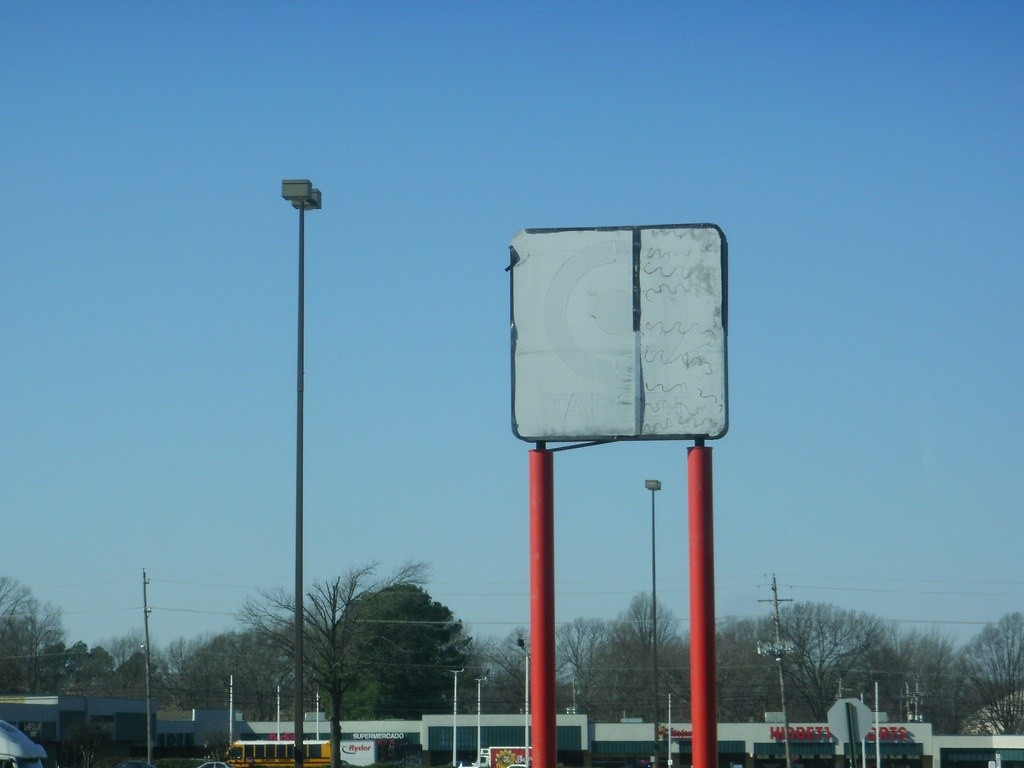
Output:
[222,739,332,768]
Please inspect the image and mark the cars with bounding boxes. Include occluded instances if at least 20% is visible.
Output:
[173,760,230,768]
[114,760,157,767]
[447,761,475,768]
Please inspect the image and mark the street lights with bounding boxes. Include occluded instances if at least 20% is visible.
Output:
[474,676,488,759]
[644,478,662,767]
[449,668,466,768]
[281,179,321,767]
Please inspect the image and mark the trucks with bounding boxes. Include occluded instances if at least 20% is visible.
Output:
[476,747,533,768]
